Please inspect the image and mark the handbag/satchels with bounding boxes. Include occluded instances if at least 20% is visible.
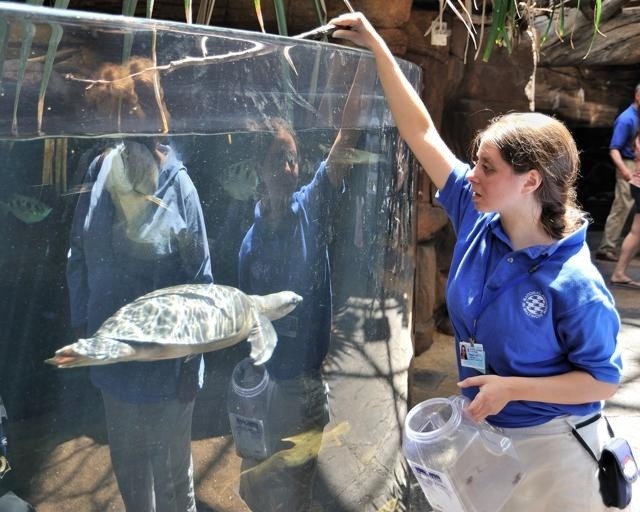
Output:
[597,434,638,509]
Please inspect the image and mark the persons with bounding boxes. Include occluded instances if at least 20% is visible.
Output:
[611,105,640,289]
[66,57,215,512]
[330,9,623,512]
[238,51,375,511]
[596,84,640,263]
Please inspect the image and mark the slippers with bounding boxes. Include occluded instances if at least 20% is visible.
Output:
[610,276,640,289]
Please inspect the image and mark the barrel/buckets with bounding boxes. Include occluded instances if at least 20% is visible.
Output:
[226,356,288,462]
[402,393,527,512]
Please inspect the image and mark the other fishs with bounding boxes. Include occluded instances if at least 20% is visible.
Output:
[212,157,259,201]
[329,144,387,166]
[0,191,51,226]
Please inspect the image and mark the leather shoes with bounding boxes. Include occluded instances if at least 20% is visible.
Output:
[595,251,618,261]
